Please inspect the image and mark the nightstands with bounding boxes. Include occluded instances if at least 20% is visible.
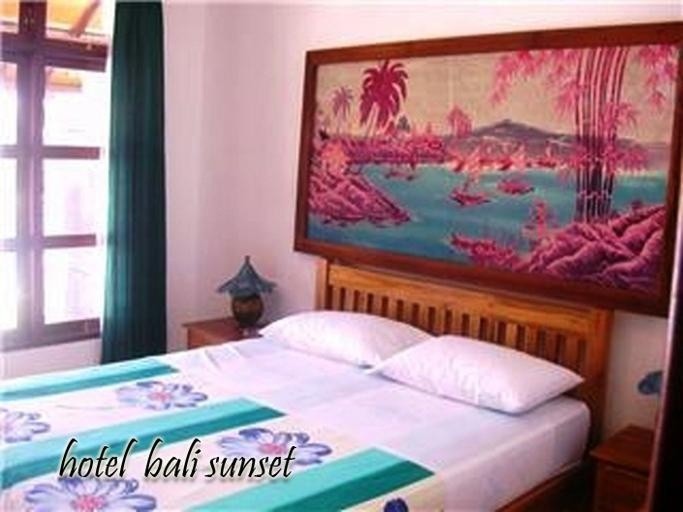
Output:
[588,423,656,512]
[181,315,269,351]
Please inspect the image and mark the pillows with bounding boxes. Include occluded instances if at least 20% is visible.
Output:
[363,334,585,416]
[257,310,437,368]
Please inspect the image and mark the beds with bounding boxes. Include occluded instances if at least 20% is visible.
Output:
[1,256,612,511]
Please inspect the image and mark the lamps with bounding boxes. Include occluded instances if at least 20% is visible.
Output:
[638,370,663,433]
[214,256,278,338]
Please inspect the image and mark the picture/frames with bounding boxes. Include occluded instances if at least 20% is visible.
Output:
[293,19,683,319]
